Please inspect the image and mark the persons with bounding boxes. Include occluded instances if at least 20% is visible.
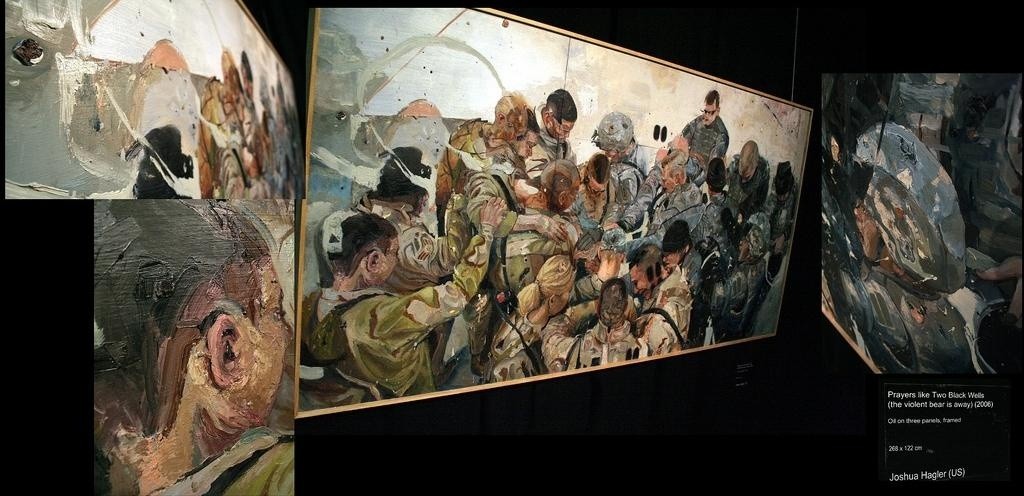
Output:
[181,47,302,197]
[299,83,797,395]
[94,198,296,496]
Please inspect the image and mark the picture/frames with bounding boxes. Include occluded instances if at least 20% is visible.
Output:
[295,7,815,428]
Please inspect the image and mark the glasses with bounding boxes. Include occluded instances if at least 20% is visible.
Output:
[591,184,606,193]
[574,192,580,200]
[704,109,717,116]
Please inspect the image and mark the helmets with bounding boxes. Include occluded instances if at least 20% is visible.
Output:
[597,111,633,151]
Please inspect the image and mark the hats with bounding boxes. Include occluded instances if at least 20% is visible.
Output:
[662,219,690,253]
[379,147,432,192]
